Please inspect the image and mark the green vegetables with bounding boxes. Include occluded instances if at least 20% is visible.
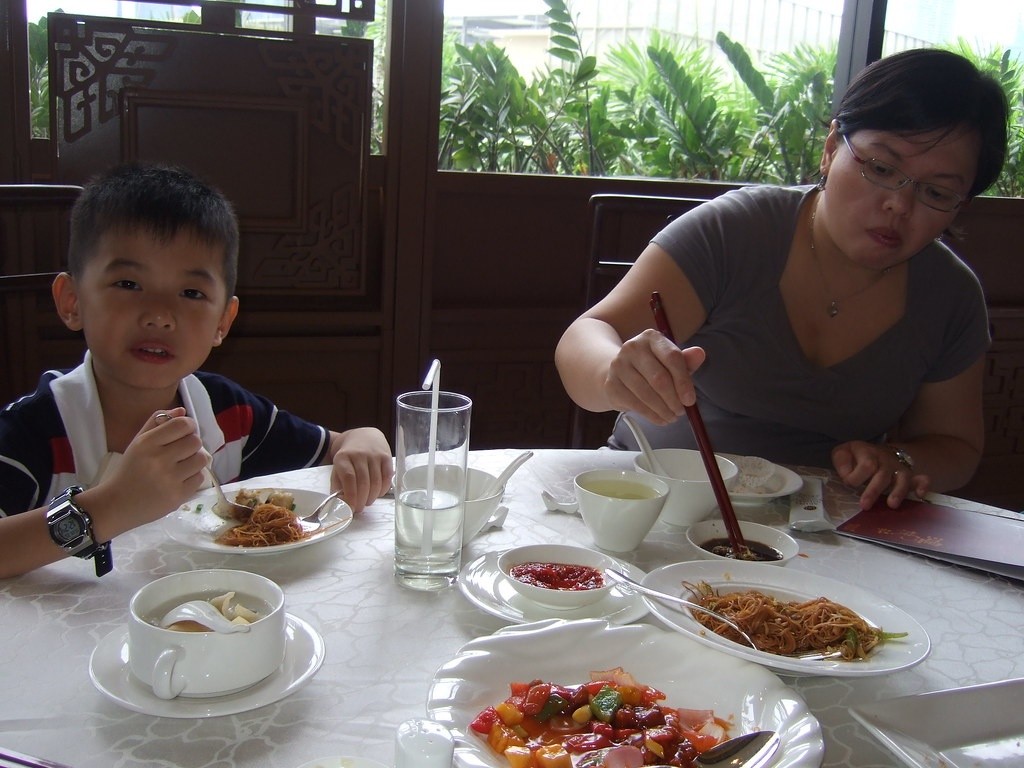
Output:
[842,628,908,657]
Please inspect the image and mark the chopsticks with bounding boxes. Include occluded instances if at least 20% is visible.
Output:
[650,291,746,555]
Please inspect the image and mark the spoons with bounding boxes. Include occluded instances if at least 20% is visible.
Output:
[297,489,344,533]
[156,414,255,521]
[160,601,250,634]
[635,731,781,768]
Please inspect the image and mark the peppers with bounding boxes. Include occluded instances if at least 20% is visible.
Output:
[472,669,725,768]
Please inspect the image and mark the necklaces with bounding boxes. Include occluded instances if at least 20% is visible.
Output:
[807,208,891,317]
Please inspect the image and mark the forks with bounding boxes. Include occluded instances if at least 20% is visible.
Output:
[605,569,842,662]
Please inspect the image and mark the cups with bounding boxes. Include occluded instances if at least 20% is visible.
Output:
[129,569,285,700]
[394,392,473,592]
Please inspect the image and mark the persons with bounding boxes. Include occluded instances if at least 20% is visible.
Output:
[552,44,1014,511]
[0,158,398,579]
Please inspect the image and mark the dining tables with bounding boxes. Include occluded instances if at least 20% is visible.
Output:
[0,448,1024,768]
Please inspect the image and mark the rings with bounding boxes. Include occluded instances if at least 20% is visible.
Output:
[892,470,901,478]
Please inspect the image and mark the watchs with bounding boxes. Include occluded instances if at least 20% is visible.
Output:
[43,484,115,578]
[882,441,915,471]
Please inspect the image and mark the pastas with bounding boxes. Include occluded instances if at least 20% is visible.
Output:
[682,582,880,657]
[217,503,309,547]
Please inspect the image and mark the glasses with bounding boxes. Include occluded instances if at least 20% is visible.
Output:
[841,133,966,212]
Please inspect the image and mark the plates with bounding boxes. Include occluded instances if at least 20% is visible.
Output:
[847,678,1024,768]
[427,619,824,768]
[713,451,804,505]
[162,488,353,557]
[457,545,651,625]
[638,558,932,679]
[87,612,325,719]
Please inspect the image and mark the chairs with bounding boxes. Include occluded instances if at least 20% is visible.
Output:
[569,193,711,448]
[1,185,83,408]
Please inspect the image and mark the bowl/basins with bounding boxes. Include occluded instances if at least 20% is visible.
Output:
[390,464,505,548]
[734,456,776,487]
[685,518,800,568]
[574,469,670,553]
[632,448,738,527]
[497,546,622,610]
